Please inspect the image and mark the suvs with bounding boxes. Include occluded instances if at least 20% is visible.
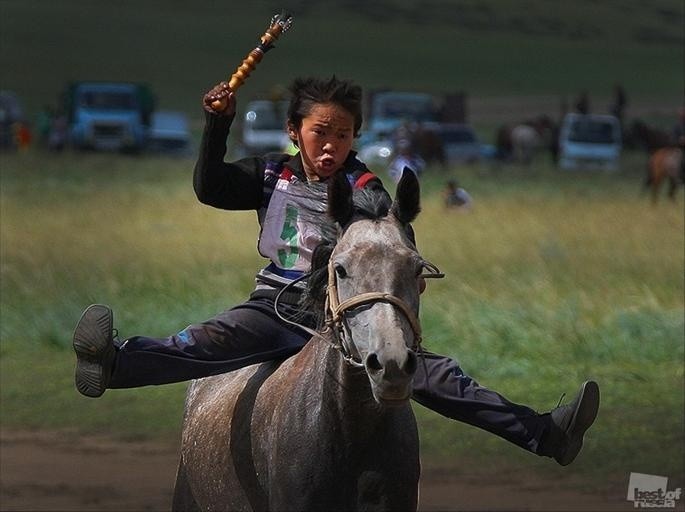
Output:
[57,76,628,188]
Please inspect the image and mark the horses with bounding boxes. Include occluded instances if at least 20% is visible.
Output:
[639,141,685,204]
[170,164,426,512]
[624,120,678,197]
[491,115,557,176]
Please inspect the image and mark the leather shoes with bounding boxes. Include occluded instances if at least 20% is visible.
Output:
[548,380,600,467]
[72,303,119,398]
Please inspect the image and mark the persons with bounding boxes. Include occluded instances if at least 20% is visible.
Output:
[440,178,473,212]
[72,79,599,467]
[577,88,589,116]
[611,80,630,121]
